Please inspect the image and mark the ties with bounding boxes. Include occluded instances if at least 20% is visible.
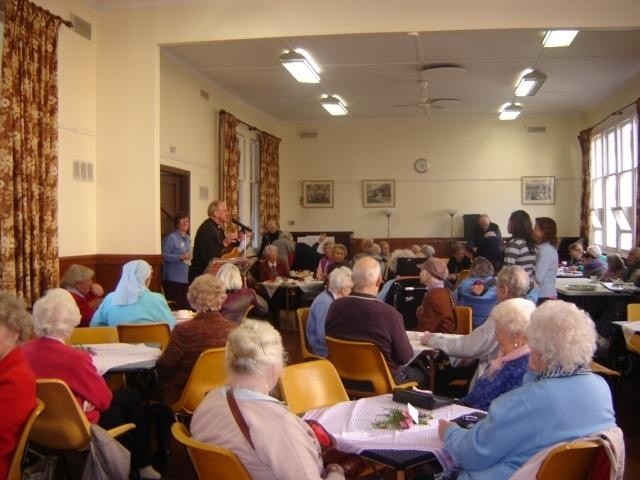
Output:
[271,265,276,275]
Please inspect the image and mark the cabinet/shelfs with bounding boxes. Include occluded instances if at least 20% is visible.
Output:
[289,229,354,258]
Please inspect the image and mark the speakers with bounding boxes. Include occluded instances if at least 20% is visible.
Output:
[462,214,481,246]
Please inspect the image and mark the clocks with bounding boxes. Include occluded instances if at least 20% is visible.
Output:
[414,158,430,173]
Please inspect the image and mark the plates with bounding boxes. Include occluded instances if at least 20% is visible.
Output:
[564,283,597,291]
[176,317,195,321]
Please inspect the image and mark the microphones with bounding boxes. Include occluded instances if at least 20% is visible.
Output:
[232,218,252,232]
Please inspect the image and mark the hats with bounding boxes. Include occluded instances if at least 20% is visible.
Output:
[416,257,449,281]
[60,263,95,286]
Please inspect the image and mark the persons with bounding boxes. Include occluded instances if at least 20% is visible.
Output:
[306,266,355,357]
[144,273,237,402]
[357,237,501,335]
[158,200,347,317]
[1,287,39,480]
[31,286,163,478]
[306,184,330,204]
[565,239,640,343]
[325,257,425,390]
[190,319,349,479]
[92,260,176,330]
[60,264,104,328]
[420,263,536,359]
[465,210,560,305]
[461,296,540,411]
[438,300,608,479]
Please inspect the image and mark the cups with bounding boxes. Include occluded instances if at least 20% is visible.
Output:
[590,274,598,283]
[177,309,191,318]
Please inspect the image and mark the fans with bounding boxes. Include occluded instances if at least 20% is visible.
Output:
[392,81,444,116]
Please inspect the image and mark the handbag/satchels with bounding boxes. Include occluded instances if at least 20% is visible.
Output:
[450,412,487,429]
[391,388,455,410]
[321,448,364,478]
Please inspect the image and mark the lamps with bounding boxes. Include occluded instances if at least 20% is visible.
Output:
[497,103,523,122]
[447,207,458,250]
[513,71,547,98]
[541,29,579,49]
[319,96,350,117]
[382,207,393,246]
[279,49,322,86]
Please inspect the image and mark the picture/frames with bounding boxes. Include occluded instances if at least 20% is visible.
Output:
[521,175,556,204]
[299,179,335,209]
[362,178,396,209]
